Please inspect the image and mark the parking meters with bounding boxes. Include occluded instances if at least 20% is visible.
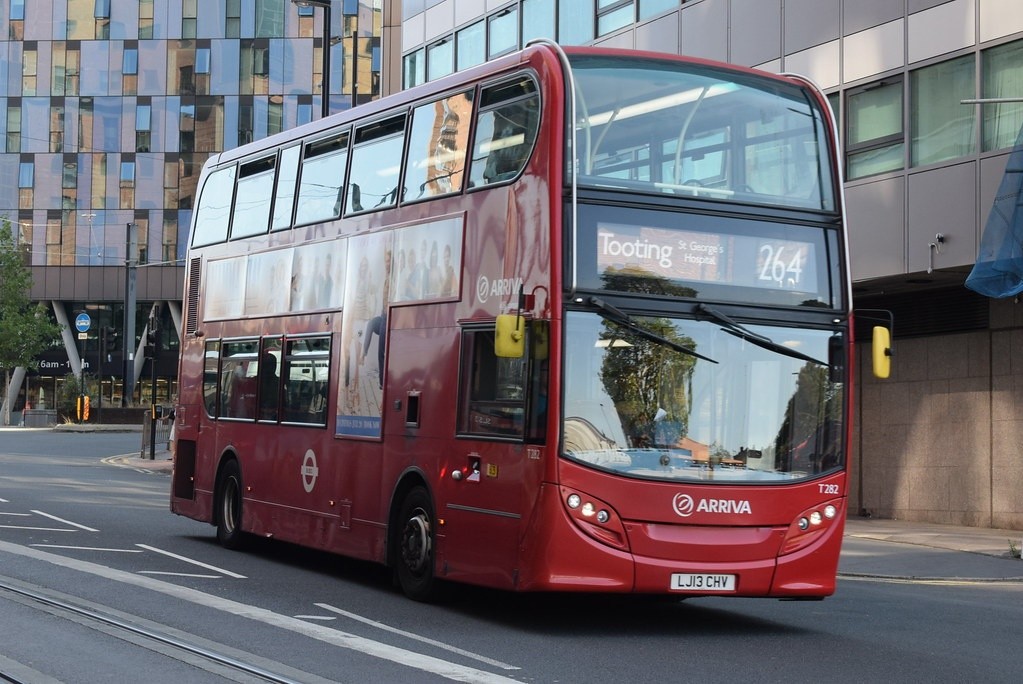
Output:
[150,404,163,461]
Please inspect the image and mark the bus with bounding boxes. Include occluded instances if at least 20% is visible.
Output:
[168,37,898,604]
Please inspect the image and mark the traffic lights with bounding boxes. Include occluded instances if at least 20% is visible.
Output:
[104,326,117,354]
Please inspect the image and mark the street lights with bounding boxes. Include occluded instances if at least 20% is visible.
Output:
[290,0,331,119]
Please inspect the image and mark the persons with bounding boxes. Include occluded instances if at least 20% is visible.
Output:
[247,353,288,408]
[159,407,177,463]
[307,239,458,413]
